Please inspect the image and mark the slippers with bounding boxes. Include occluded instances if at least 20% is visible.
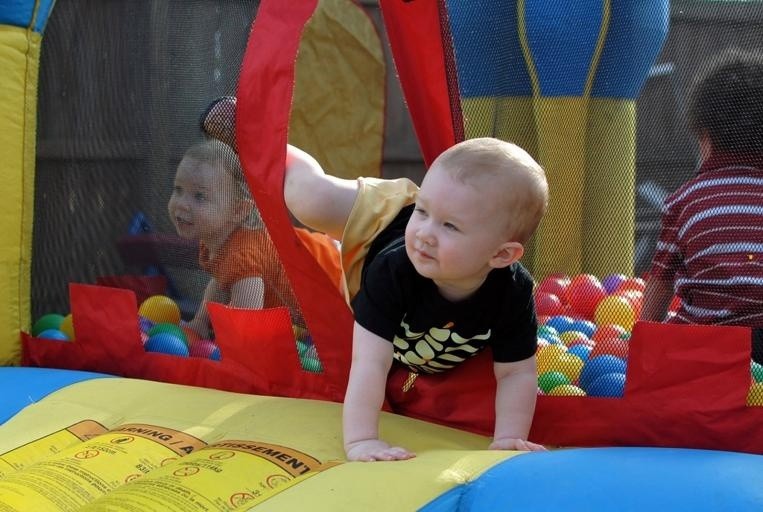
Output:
[202,100,237,149]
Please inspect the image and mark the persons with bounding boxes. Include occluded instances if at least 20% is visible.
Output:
[194,94,552,462]
[165,135,344,347]
[637,48,763,366]
